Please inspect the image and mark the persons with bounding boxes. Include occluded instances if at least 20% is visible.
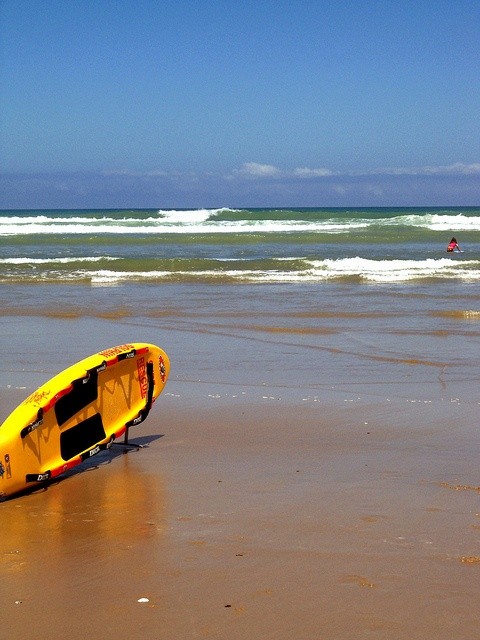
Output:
[447,238,460,252]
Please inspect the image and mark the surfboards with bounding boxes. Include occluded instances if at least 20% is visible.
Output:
[454,251,464,254]
